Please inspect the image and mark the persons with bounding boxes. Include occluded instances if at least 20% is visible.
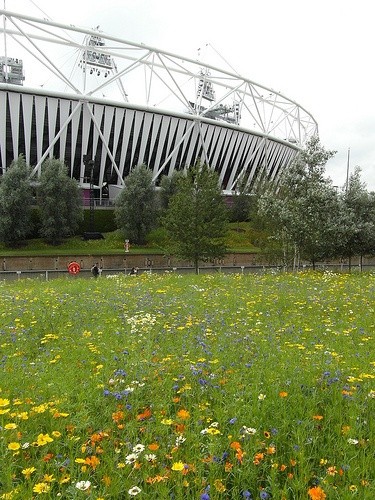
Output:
[91,265,95,273]
[131,267,134,274]
[94,263,99,276]
